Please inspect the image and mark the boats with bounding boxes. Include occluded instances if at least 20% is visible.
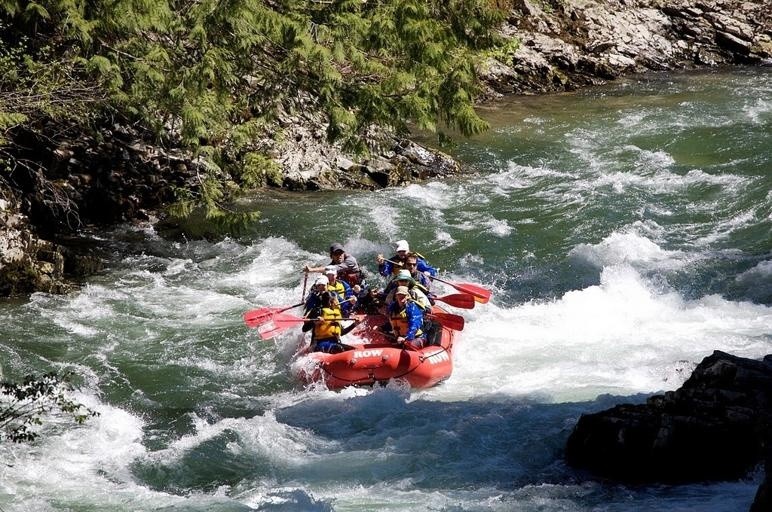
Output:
[297,304,454,388]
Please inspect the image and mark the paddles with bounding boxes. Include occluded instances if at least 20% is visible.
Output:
[381,258,491,304]
[273,313,358,329]
[368,327,421,352]
[244,288,355,329]
[427,293,475,310]
[257,298,354,341]
[425,312,464,331]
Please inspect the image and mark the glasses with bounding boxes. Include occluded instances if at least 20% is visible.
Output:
[405,263,416,267]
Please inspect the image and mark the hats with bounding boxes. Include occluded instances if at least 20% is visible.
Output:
[330,242,344,252]
[396,269,411,280]
[396,239,409,251]
[324,265,337,275]
[315,275,329,286]
[395,285,409,295]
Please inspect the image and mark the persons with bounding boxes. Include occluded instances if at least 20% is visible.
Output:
[302,290,365,353]
[372,285,437,350]
[301,239,438,331]
